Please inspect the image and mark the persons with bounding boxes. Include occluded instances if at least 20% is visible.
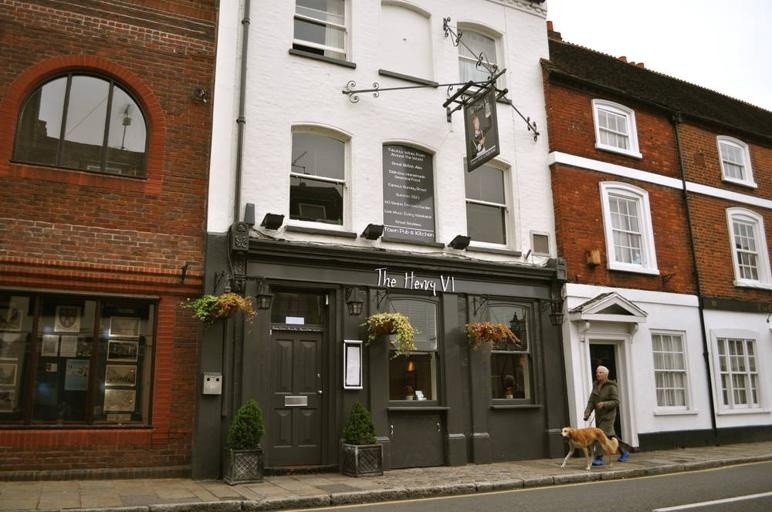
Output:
[584,365,630,466]
[471,110,492,158]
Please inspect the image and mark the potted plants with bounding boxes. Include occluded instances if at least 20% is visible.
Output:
[222,398,265,486]
[359,311,423,360]
[464,321,522,350]
[338,396,384,477]
[180,292,257,336]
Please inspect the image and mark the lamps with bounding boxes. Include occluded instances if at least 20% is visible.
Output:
[447,234,471,250]
[260,213,285,231]
[345,286,364,316]
[255,278,275,311]
[360,224,385,240]
[549,299,564,326]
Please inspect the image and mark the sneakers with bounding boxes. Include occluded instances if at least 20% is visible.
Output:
[617,451,630,462]
[591,456,605,465]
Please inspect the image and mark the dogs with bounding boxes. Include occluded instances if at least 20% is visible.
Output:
[560,426,619,470]
